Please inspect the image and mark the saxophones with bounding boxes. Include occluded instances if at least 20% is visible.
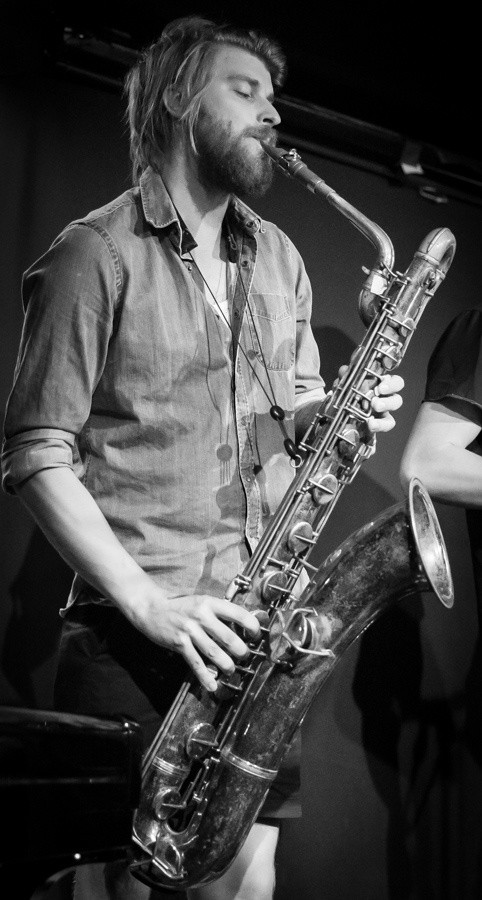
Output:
[127,137,457,892]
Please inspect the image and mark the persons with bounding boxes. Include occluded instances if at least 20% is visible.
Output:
[0,14,405,900]
[399,302,482,623]
